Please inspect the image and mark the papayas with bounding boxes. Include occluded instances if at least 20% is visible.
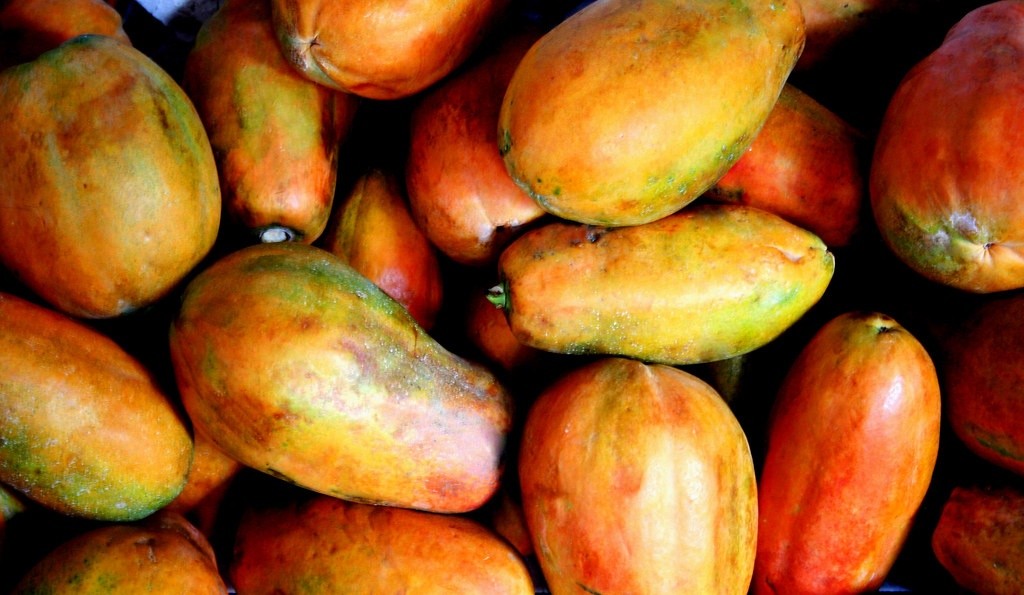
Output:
[0,0,1024,595]
